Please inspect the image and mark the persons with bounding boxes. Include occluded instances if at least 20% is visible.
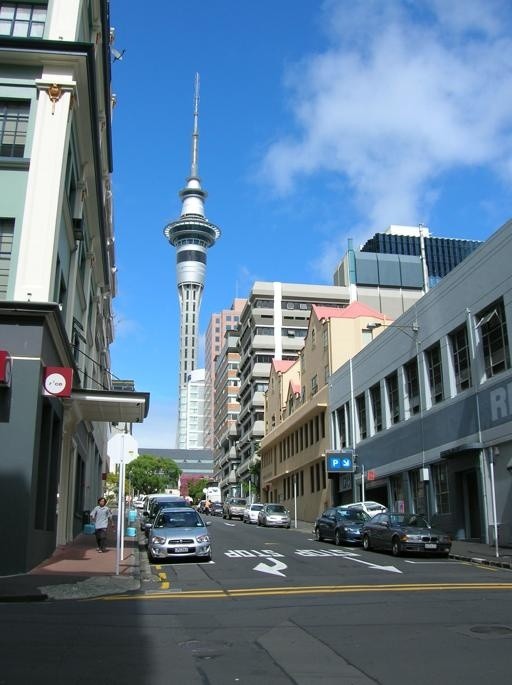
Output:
[204,500,212,516]
[89,497,114,554]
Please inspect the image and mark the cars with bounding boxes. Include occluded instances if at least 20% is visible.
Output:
[135,493,193,537]
[144,507,212,562]
[258,503,291,529]
[211,503,224,516]
[197,500,213,514]
[314,501,389,545]
[360,512,452,557]
[243,504,264,523]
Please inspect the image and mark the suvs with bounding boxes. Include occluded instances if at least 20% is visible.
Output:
[222,498,247,521]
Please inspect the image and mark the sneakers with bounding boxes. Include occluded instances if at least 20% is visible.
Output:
[97,546,103,553]
